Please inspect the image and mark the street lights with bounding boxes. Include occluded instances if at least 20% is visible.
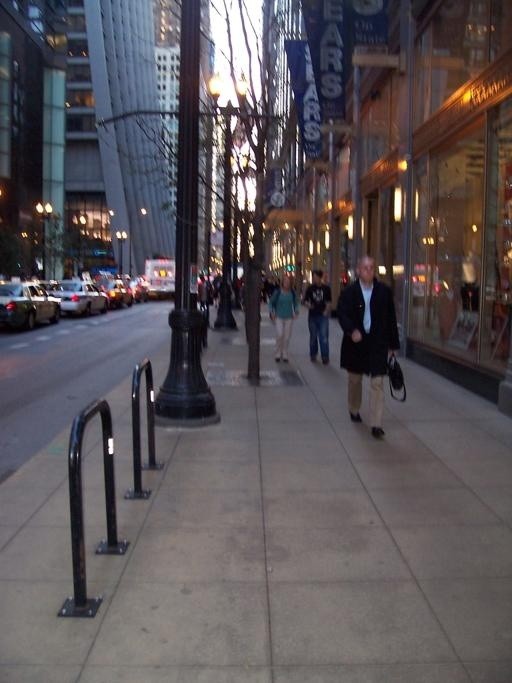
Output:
[115,230,129,278]
[35,200,53,280]
[204,72,257,327]
[130,206,150,277]
[72,214,87,277]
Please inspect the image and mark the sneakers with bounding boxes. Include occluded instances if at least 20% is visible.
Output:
[275,354,289,362]
[351,413,361,423]
[372,427,384,437]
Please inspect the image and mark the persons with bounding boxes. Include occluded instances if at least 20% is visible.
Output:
[270,277,299,361]
[212,271,280,311]
[337,255,405,436]
[300,269,332,364]
[198,273,213,312]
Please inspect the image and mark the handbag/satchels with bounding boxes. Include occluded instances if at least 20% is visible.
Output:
[389,355,406,401]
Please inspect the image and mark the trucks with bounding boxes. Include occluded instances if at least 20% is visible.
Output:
[144,255,176,298]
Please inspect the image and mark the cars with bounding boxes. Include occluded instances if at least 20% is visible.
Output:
[0,273,153,335]
[304,262,453,330]
